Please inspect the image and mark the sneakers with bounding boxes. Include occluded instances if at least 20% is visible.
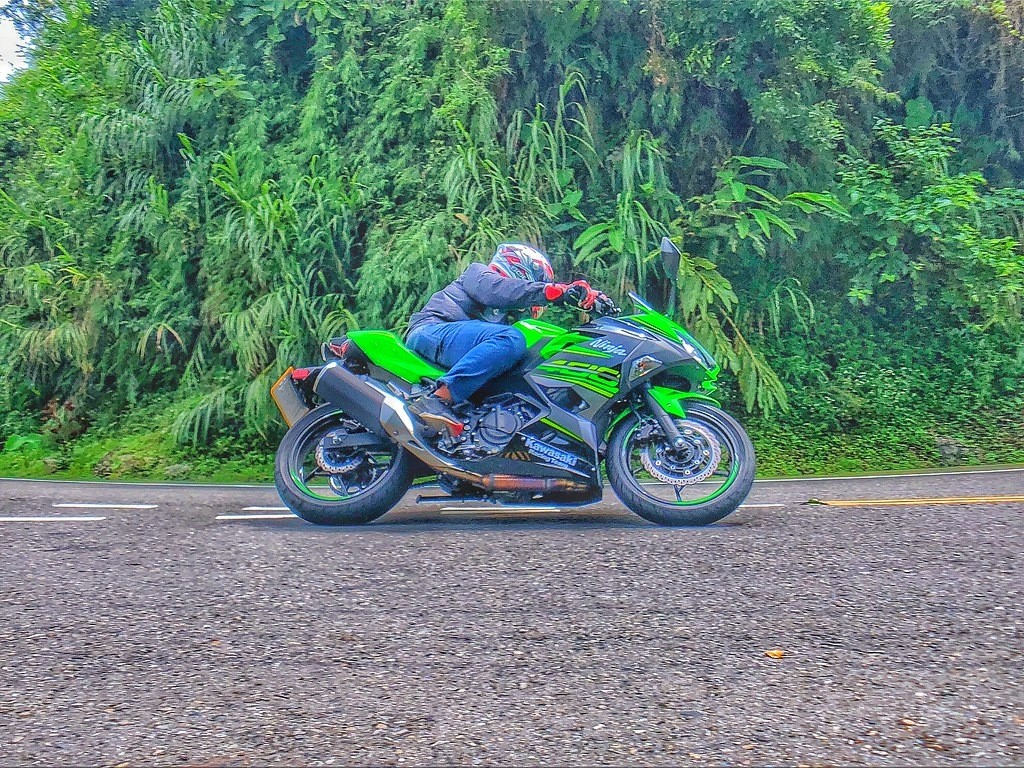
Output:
[408,394,462,436]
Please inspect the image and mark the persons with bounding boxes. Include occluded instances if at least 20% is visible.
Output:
[405,243,610,437]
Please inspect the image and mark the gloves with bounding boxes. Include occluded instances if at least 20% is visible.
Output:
[543,280,602,307]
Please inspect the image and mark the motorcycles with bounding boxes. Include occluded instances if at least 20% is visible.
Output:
[268,287,756,526]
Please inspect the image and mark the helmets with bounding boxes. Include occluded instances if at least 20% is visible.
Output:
[488,243,554,317]
